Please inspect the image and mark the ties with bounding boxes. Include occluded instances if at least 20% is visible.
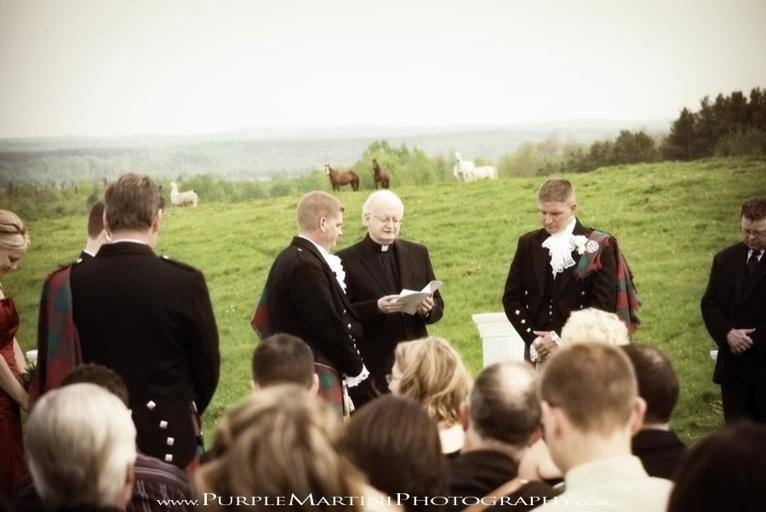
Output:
[747,249,761,264]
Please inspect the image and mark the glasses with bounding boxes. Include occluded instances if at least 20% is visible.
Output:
[739,227,766,237]
[371,214,403,223]
[386,374,405,384]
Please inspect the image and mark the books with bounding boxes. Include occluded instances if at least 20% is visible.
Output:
[395,280,443,316]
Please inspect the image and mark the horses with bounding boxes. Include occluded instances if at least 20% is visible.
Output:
[323,163,359,191]
[159,185,165,214]
[452,150,499,183]
[170,182,198,208]
[371,158,390,190]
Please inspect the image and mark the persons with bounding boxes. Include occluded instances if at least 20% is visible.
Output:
[670,415,765,512]
[335,189,448,414]
[500,178,642,368]
[0,208,40,512]
[248,188,369,420]
[1,306,689,512]
[36,172,219,479]
[35,200,112,399]
[699,197,765,423]
